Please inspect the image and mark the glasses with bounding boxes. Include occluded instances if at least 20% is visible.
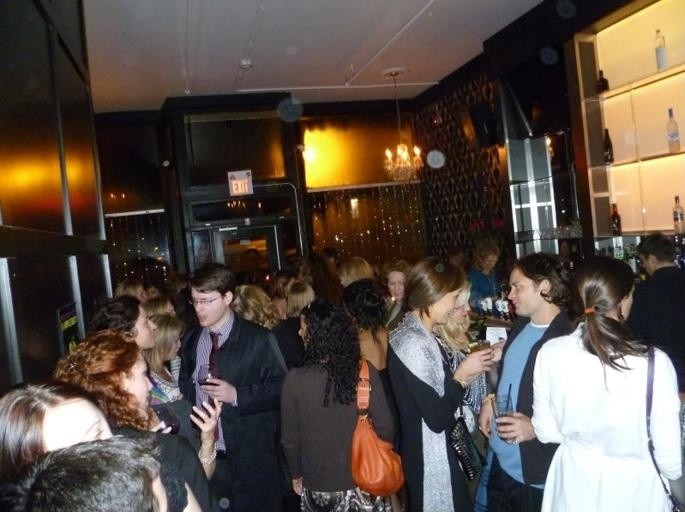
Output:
[190,294,221,306]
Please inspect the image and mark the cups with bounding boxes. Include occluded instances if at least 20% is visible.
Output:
[490,394,518,444]
[468,339,491,351]
[196,363,218,387]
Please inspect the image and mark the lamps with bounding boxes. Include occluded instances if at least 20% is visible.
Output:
[381,66,423,181]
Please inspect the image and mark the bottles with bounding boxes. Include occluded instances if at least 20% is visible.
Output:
[609,202,623,236]
[602,127,615,164]
[652,29,668,71]
[671,195,685,231]
[611,236,685,277]
[480,296,516,320]
[663,108,682,156]
[596,69,610,94]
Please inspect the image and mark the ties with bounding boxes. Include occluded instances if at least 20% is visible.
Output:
[207,331,221,440]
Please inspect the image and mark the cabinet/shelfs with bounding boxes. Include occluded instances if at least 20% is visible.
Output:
[563,0,685,281]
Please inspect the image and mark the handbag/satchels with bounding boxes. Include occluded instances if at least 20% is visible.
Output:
[351,414,405,496]
[449,383,487,480]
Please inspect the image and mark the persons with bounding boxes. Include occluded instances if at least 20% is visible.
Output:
[1,231,684,512]
